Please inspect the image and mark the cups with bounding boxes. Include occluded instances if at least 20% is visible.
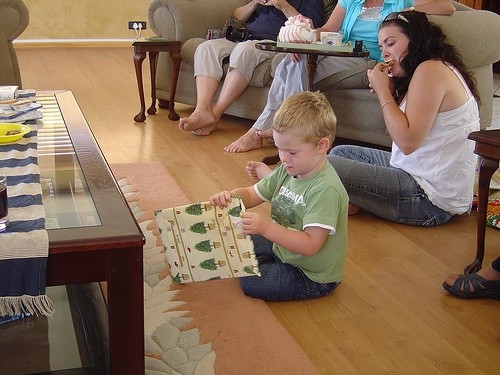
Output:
[0,172,9,232]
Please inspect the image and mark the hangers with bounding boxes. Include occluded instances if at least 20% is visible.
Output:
[40,162,319,375]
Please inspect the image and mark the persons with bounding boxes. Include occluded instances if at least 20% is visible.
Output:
[222,0,457,155]
[205,90,352,302]
[177,0,327,137]
[442,253,500,302]
[244,8,482,228]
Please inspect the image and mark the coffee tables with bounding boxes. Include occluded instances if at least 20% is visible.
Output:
[133,36,182,123]
[0,91,148,375]
[253,39,370,163]
[463,128,500,279]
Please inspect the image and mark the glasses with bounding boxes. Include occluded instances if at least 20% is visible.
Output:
[381,12,409,24]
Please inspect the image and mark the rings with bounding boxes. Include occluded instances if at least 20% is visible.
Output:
[271,0,274,2]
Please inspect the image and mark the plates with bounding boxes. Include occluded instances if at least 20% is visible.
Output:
[0,122,31,142]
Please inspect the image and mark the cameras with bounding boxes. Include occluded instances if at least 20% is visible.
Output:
[226,26,254,42]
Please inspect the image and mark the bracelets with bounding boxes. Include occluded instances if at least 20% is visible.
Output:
[380,98,395,110]
[410,5,416,12]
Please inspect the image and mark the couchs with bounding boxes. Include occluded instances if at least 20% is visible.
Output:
[148,1,500,160]
[0,0,30,91]
